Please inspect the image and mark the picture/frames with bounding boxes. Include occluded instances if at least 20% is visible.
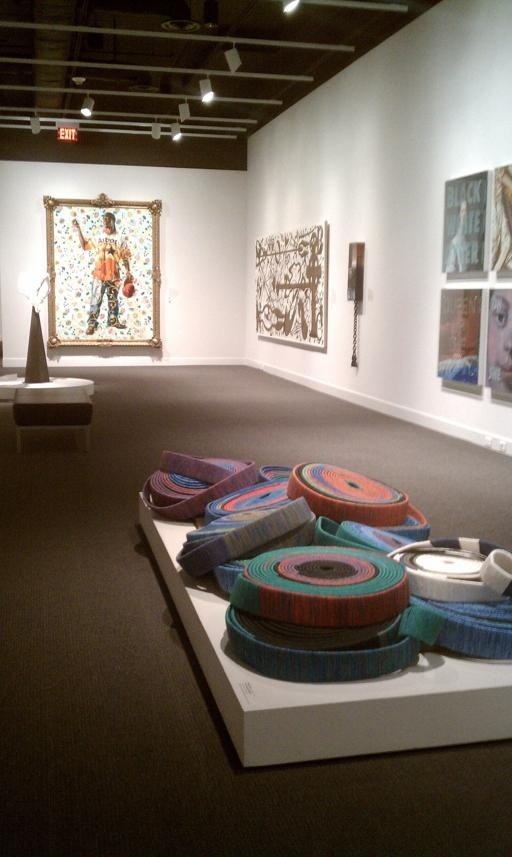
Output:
[438,288,488,386]
[43,192,163,352]
[442,169,492,274]
[256,219,328,349]
[485,288,512,394]
[491,163,512,272]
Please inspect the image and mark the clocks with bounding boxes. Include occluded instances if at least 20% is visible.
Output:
[347,242,365,367]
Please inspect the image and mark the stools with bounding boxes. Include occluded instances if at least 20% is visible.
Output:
[13,386,94,454]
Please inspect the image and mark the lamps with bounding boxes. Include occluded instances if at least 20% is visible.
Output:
[151,117,161,140]
[199,74,215,104]
[30,111,41,135]
[224,40,248,84]
[282,0,301,14]
[178,97,190,122]
[171,119,182,141]
[81,93,95,117]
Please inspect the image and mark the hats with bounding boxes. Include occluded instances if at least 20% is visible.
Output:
[100,211,115,219]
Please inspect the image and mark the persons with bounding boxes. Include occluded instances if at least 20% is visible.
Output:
[489,291,512,389]
[71,212,133,335]
[446,200,467,272]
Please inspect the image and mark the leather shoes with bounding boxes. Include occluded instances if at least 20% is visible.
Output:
[107,318,127,329]
[85,325,95,335]
